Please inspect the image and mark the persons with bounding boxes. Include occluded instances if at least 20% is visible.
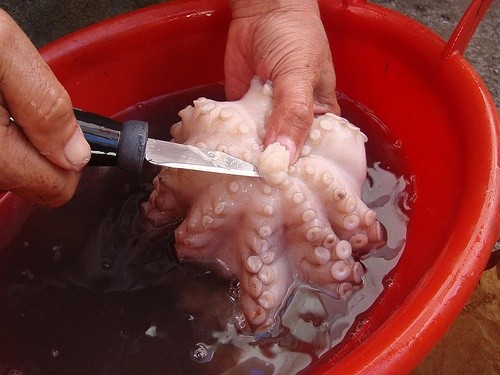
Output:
[1,0,343,211]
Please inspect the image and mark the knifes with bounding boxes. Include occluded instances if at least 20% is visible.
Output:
[10,105,268,179]
